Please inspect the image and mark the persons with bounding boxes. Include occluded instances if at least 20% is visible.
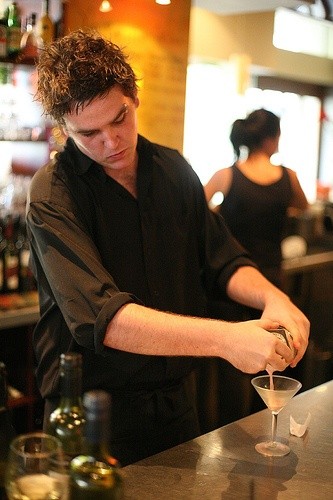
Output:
[22,31,309,461]
[203,108,330,323]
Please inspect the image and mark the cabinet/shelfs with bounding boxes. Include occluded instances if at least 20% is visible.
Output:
[0,245,333,409]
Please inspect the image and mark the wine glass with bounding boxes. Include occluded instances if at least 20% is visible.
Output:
[251,375,303,458]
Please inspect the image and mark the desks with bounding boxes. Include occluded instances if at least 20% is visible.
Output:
[118,380,333,500]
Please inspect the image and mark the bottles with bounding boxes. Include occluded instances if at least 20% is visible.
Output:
[42,352,90,500]
[68,391,125,500]
[0,0,57,64]
[265,327,299,370]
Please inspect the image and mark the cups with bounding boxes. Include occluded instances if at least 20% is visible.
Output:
[4,433,66,500]
